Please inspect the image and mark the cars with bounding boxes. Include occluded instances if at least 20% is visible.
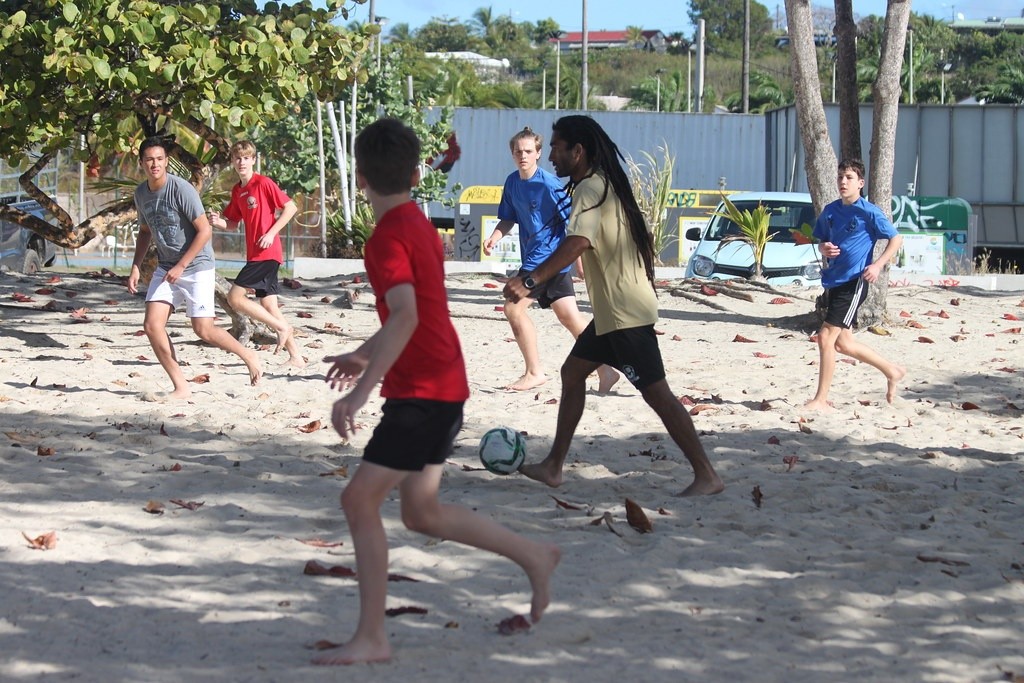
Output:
[682,192,833,283]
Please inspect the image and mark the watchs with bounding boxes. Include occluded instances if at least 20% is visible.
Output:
[520,271,537,291]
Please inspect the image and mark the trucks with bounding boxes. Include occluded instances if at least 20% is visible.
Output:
[0,149,59,274]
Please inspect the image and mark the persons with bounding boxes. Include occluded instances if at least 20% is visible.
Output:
[494,113,727,502]
[482,124,620,395]
[309,114,565,667]
[205,138,308,369]
[797,156,906,408]
[124,136,263,396]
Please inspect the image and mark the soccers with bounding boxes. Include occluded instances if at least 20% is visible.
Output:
[479,426,527,475]
